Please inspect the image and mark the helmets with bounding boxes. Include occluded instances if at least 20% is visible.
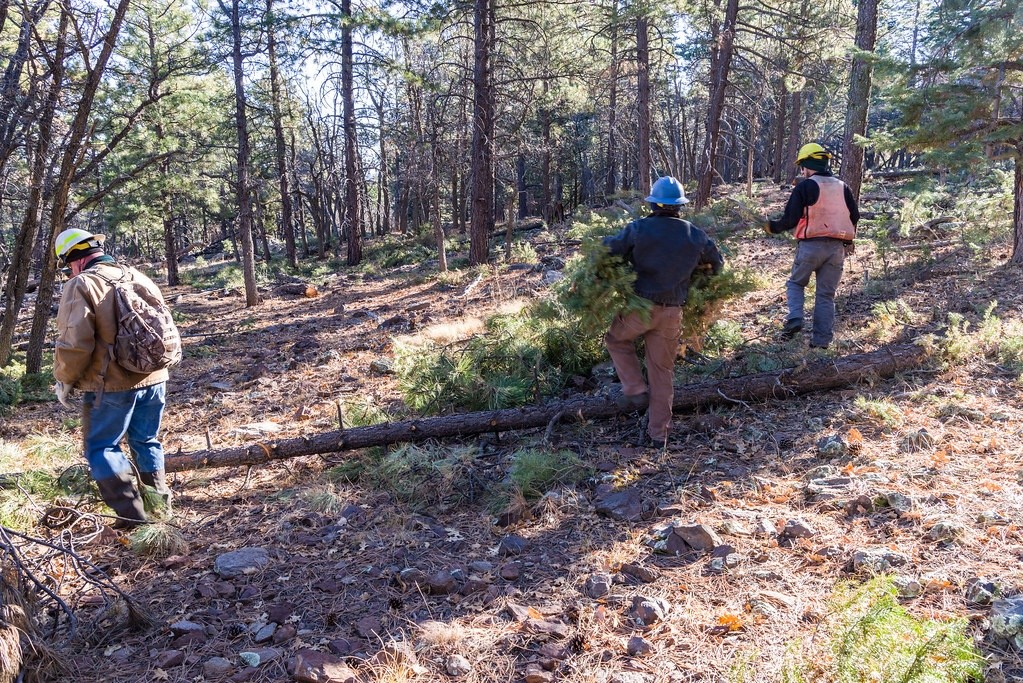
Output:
[644,175,689,205]
[54,227,106,260]
[794,142,831,164]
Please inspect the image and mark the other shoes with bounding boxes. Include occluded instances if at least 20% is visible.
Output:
[98,469,172,531]
[782,319,802,342]
[646,433,664,449]
[632,394,646,404]
[809,340,827,349]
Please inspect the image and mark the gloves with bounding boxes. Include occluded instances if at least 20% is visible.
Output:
[56,382,71,408]
[763,221,773,235]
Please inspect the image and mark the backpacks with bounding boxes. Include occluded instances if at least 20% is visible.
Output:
[83,265,182,374]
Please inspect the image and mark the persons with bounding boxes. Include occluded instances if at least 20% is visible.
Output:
[54,227,170,534]
[764,142,860,350]
[602,176,722,451]
